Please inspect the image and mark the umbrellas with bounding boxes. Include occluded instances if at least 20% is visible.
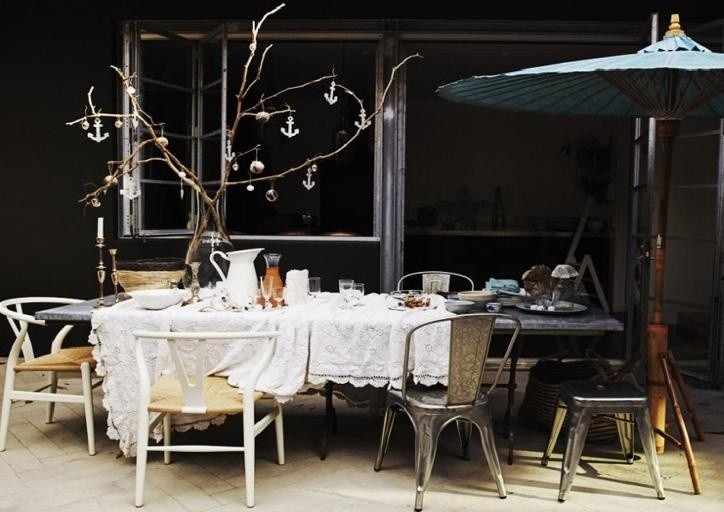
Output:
[434,12,724,451]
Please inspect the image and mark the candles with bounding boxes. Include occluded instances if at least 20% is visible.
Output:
[97,218,104,238]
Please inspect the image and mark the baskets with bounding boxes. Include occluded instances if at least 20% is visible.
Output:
[115,257,186,296]
[516,358,639,443]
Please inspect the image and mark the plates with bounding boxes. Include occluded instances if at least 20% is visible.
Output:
[388,303,438,311]
[496,294,523,307]
[515,299,588,314]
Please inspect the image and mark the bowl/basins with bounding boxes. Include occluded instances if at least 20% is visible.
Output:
[129,290,185,310]
[444,300,475,314]
[457,290,498,311]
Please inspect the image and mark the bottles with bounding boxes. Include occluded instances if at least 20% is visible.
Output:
[258,253,284,309]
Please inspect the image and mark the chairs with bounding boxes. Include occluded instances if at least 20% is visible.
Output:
[0,297,102,457]
[133,327,286,508]
[396,271,474,294]
[375,312,521,512]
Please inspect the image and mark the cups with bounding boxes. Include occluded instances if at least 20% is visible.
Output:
[308,277,321,295]
[485,302,503,312]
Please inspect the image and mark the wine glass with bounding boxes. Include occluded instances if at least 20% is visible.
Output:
[272,287,285,311]
[260,275,273,312]
[338,279,355,311]
[352,283,366,307]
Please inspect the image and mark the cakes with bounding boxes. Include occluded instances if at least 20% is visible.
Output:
[398,293,431,310]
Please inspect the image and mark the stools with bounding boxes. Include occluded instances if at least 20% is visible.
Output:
[542,380,666,502]
